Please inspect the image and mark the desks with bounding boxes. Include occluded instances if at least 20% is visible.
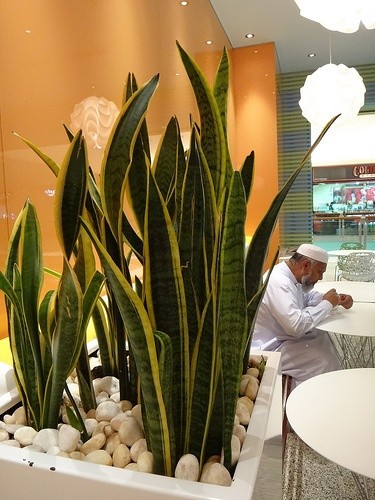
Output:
[298,280,374,373]
[324,249,375,266]
[282,367,375,500]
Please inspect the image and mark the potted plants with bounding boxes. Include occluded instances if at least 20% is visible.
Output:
[0,36,346,499]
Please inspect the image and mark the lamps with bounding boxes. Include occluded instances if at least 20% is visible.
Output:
[298,30,367,129]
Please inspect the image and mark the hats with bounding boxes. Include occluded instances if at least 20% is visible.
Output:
[296,244,329,263]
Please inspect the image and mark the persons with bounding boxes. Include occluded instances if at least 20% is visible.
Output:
[250,243,354,382]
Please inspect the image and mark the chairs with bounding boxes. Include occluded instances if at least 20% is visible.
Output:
[338,251,375,284]
[335,242,366,276]
